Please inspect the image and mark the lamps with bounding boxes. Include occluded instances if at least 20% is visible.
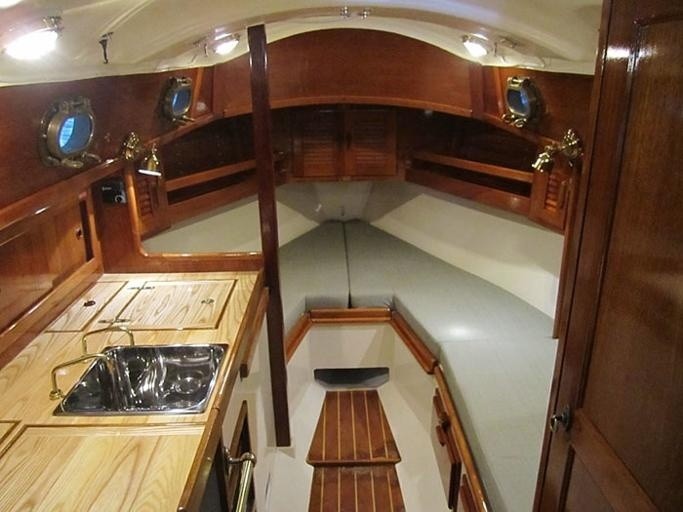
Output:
[120,129,162,179]
[531,125,584,175]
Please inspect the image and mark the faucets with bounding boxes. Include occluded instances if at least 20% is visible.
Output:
[49,353,115,401]
[82,325,135,363]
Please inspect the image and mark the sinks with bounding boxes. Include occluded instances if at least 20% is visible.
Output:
[53,343,229,417]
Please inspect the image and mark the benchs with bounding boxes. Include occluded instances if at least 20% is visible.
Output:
[278,215,560,512]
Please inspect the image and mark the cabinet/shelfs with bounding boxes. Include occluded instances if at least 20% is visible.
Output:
[456,469,479,512]
[428,383,462,512]
